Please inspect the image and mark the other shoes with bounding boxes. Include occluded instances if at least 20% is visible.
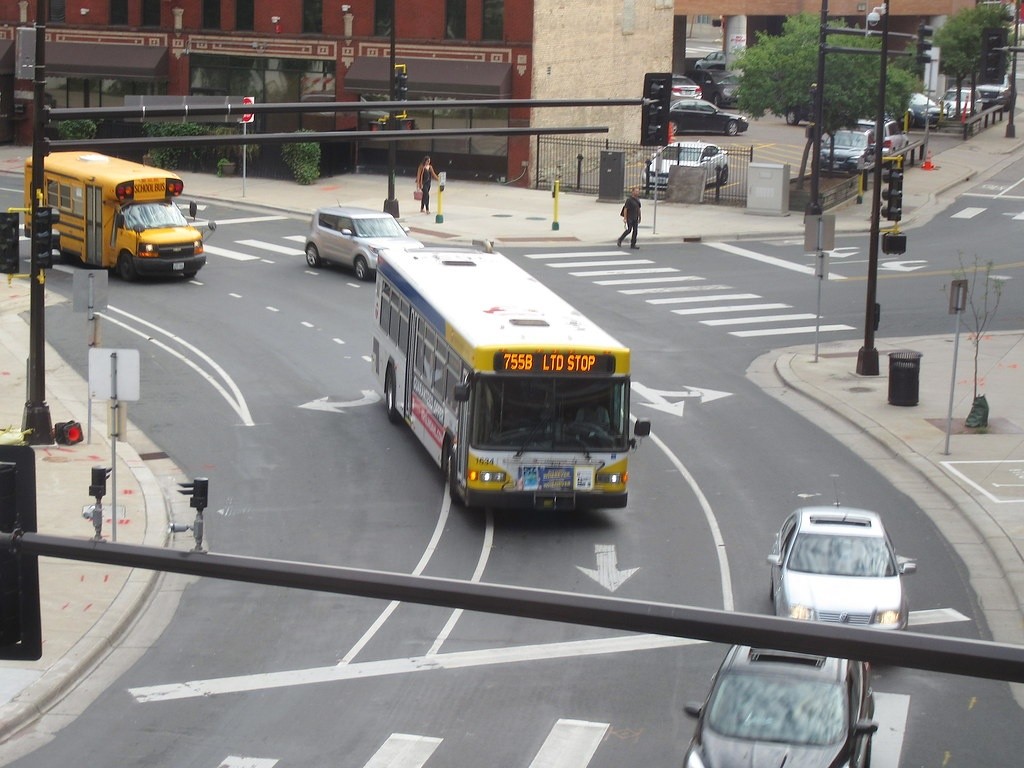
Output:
[616,239,621,247]
[631,245,640,249]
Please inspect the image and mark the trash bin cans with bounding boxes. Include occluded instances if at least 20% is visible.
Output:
[887,351,922,406]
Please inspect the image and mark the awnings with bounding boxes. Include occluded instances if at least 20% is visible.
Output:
[343,56,513,108]
[44,41,169,82]
[0,39,16,76]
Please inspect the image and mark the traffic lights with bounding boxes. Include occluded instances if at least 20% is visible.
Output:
[368,120,385,131]
[399,118,416,130]
[879,230,907,255]
[64,422,87,446]
[916,23,934,64]
[177,478,207,513]
[33,206,62,270]
[881,168,905,221]
[395,67,408,102]
[89,464,114,500]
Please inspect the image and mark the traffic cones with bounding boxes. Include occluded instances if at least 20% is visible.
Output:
[921,149,934,170]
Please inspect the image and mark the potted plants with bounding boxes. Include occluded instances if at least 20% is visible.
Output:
[142,145,156,166]
[217,156,238,177]
[280,129,321,185]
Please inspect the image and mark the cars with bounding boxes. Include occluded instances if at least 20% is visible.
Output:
[678,644,883,768]
[666,98,749,136]
[811,82,1017,179]
[670,74,703,107]
[693,51,726,70]
[684,68,751,110]
[641,141,729,192]
[766,504,919,630]
[303,205,425,281]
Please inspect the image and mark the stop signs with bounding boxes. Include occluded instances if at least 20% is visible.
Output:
[237,96,255,124]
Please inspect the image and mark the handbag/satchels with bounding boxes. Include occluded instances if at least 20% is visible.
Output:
[620,206,625,216]
[414,189,423,200]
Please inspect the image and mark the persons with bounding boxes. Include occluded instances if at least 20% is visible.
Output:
[617,186,642,250]
[415,155,439,215]
[575,389,610,441]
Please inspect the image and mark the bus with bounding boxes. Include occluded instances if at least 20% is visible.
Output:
[370,236,652,513]
[20,149,218,284]
